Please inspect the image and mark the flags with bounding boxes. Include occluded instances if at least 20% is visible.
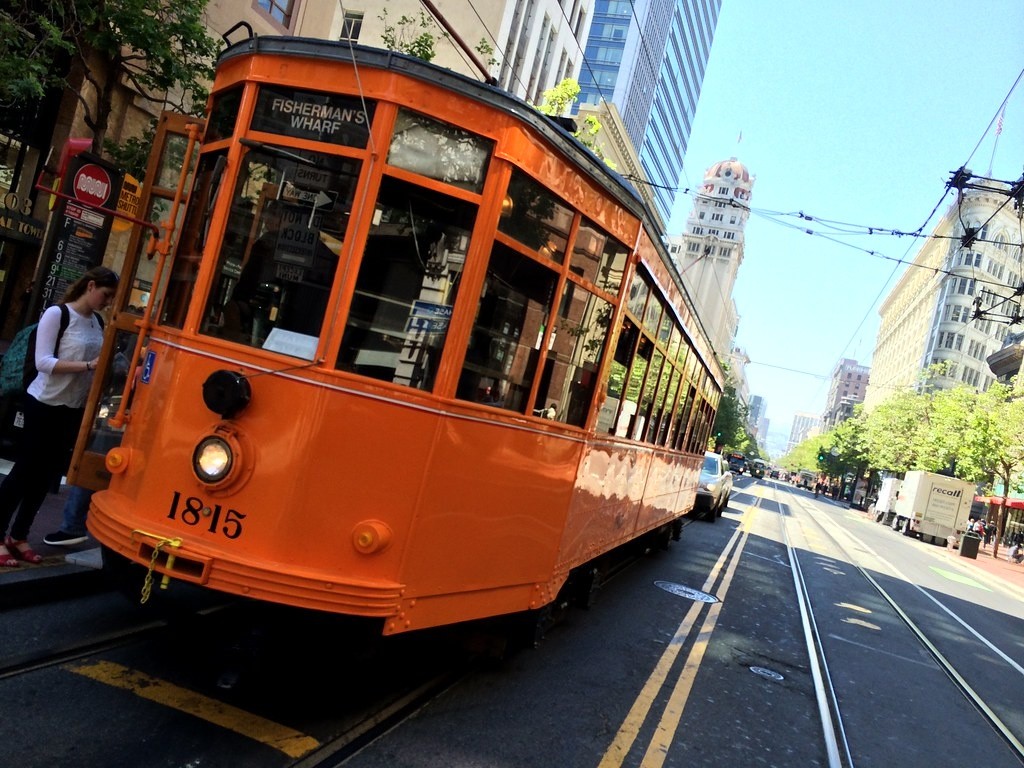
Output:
[995,107,1004,135]
[738,132,741,142]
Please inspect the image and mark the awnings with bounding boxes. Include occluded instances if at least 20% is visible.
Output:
[975,496,1024,510]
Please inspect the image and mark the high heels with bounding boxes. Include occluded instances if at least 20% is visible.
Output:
[5,535,42,564]
[0,542,21,568]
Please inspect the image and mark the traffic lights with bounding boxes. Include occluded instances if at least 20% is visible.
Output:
[717,432,722,438]
[819,454,823,461]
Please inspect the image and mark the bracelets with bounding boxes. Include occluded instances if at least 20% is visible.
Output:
[87,361,95,371]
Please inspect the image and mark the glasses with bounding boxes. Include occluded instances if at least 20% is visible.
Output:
[95,272,119,283]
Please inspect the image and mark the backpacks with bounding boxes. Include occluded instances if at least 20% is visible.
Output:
[0,305,104,403]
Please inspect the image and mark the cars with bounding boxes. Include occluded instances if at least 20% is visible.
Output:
[693,450,733,523]
[770,470,780,480]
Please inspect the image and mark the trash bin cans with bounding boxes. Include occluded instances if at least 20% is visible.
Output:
[959,531,981,559]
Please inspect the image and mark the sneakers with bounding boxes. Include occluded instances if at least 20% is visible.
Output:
[43,530,88,545]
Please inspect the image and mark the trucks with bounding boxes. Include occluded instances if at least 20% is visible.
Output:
[873,471,977,546]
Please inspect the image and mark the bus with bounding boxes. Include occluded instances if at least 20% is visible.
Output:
[60,21,728,637]
[727,452,765,479]
[796,470,817,491]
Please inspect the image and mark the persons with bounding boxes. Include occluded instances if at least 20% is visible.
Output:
[967,517,1000,548]
[803,477,839,501]
[0,267,156,568]
[1002,524,1024,563]
[221,198,339,346]
